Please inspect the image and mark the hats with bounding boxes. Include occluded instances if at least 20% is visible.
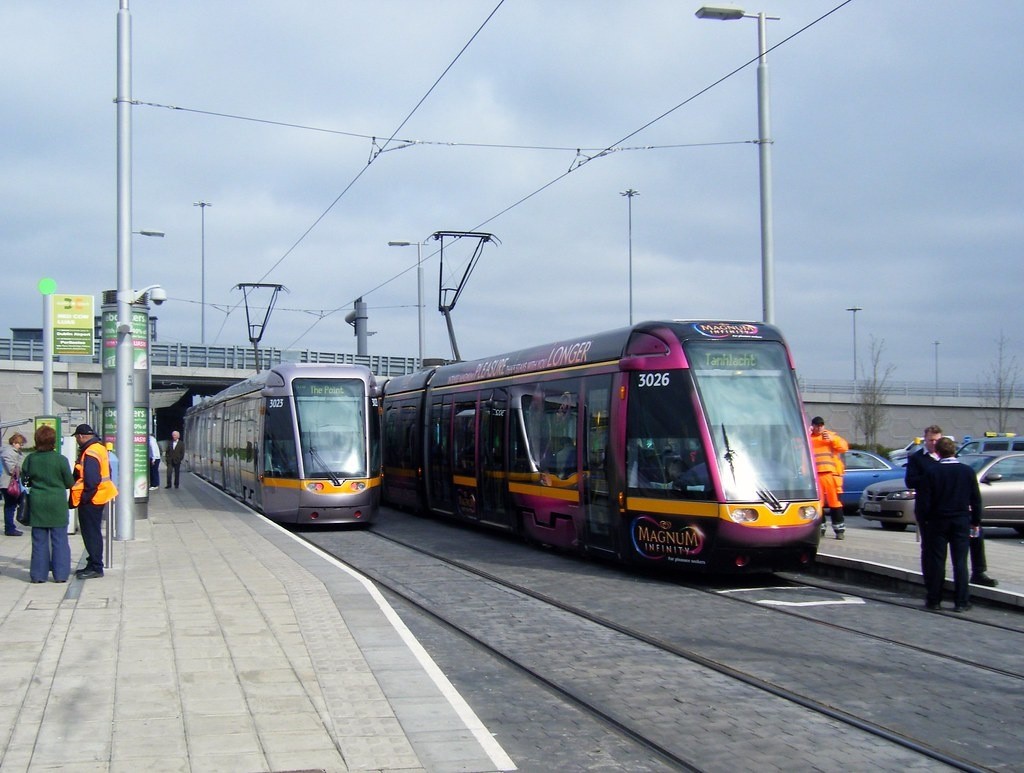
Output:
[70,424,95,437]
[812,416,824,425]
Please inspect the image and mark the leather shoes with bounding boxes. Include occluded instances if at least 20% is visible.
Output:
[75,566,104,579]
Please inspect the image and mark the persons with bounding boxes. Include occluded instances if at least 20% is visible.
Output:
[69,423,118,580]
[904,425,999,587]
[554,437,583,478]
[918,437,982,612]
[21,426,75,583]
[0,433,27,537]
[148,431,185,491]
[800,416,849,540]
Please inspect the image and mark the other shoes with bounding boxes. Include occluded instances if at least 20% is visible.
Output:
[5,529,23,536]
[836,533,845,540]
[149,486,158,491]
[953,603,973,612]
[175,485,178,488]
[925,601,941,610]
[970,572,998,586]
[165,486,172,489]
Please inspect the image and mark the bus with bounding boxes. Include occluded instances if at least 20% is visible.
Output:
[182,363,382,529]
[385,321,824,583]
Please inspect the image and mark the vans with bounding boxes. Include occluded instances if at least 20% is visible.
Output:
[956,431,1024,460]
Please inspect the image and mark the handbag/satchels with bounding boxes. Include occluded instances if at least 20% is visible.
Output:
[8,465,20,498]
[16,490,29,526]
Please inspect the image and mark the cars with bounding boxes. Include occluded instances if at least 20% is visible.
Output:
[888,435,972,468]
[858,450,1024,532]
[840,450,907,514]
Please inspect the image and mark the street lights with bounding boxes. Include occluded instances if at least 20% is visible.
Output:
[694,6,776,325]
[932,340,941,396]
[388,242,425,373]
[621,187,641,324]
[845,306,863,382]
[194,201,212,342]
